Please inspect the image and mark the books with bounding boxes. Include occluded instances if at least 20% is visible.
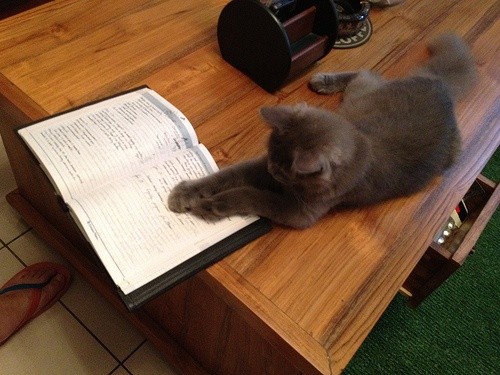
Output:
[12,84,273,313]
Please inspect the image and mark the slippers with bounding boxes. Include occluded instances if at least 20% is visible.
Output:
[0,262,70,346]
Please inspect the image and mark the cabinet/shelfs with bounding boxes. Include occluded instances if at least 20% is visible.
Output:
[0,0,500,375]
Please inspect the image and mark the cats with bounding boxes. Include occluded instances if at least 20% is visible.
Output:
[168,29,481,231]
